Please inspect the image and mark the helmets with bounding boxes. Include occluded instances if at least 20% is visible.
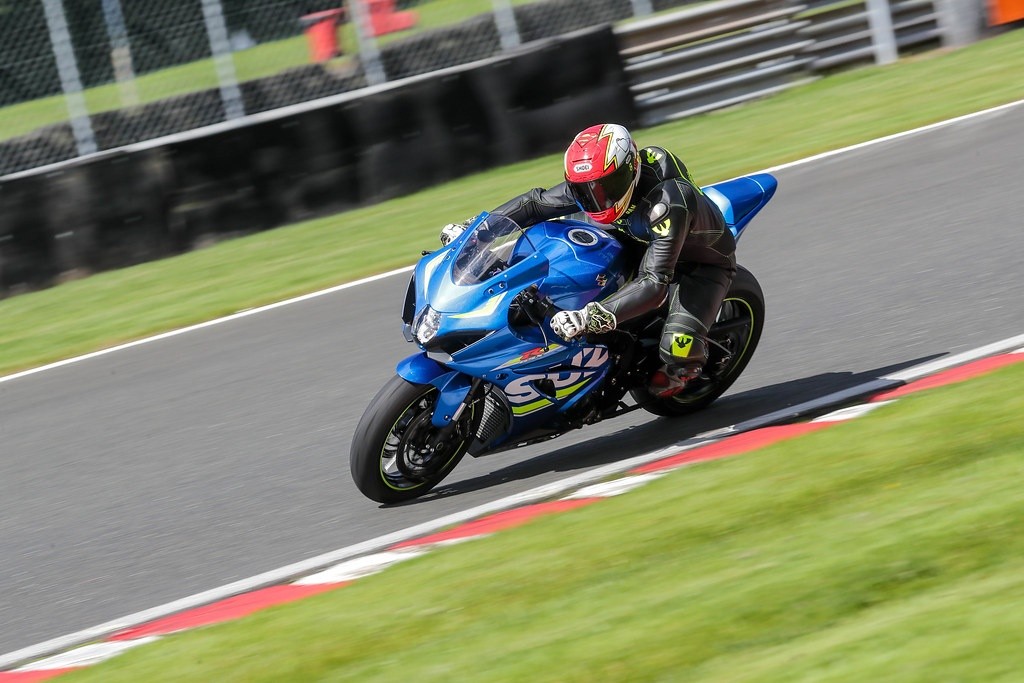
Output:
[564,124,641,224]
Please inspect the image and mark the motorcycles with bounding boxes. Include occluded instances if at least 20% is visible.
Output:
[349,170,779,505]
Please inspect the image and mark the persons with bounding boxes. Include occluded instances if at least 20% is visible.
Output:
[442,124,738,397]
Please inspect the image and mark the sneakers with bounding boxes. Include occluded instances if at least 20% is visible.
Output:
[648,363,702,397]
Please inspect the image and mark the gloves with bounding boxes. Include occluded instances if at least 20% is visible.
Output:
[440,215,490,250]
[550,302,617,342]
